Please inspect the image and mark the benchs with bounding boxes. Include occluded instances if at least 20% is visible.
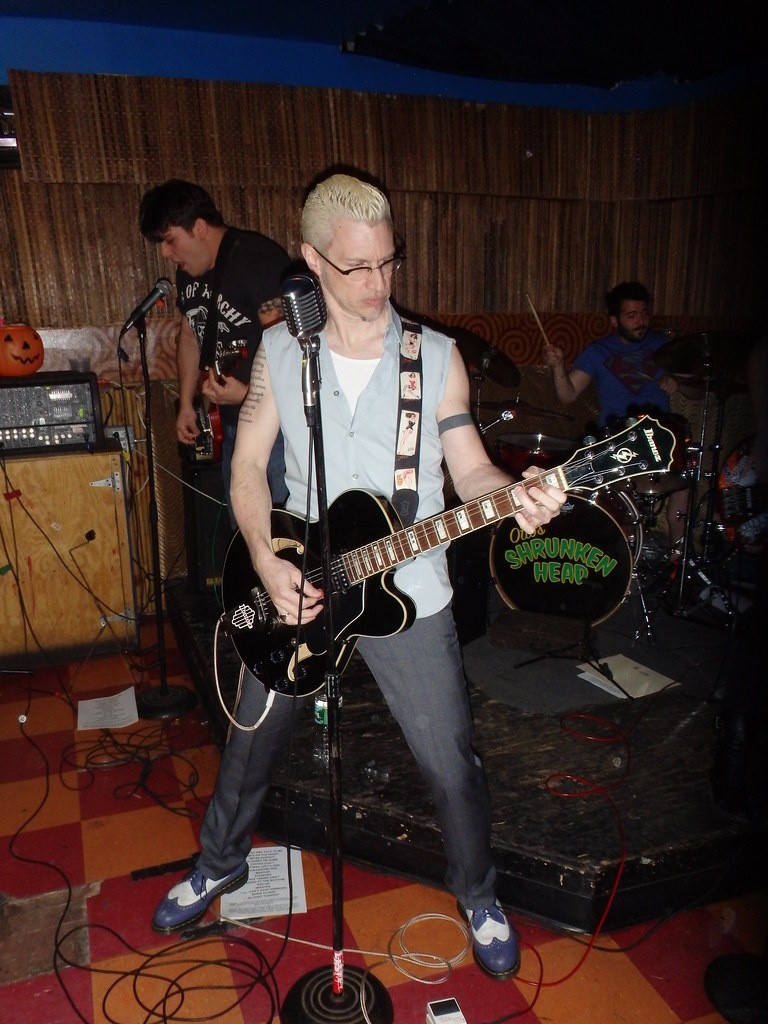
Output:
[441,367,753,506]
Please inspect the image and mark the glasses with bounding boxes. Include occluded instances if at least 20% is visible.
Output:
[313,247,406,282]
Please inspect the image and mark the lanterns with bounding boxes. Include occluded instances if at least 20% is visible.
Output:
[0,324,44,377]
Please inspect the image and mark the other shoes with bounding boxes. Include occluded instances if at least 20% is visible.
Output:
[662,542,699,578]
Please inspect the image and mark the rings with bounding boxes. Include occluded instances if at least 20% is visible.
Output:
[279,613,289,624]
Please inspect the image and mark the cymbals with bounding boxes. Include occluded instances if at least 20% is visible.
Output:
[446,326,521,388]
[655,330,753,376]
[471,402,570,421]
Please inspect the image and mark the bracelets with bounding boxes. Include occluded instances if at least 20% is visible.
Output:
[552,372,568,380]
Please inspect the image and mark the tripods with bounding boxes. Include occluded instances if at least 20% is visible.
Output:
[513,578,637,702]
[633,355,743,631]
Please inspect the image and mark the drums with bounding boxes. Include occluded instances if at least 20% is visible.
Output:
[486,485,647,628]
[616,452,695,496]
[493,431,581,484]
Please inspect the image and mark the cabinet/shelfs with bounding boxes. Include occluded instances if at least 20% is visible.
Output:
[0,439,142,674]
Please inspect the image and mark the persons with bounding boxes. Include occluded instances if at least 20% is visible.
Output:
[542,282,712,580]
[150,174,567,981]
[139,179,310,538]
[709,325,768,823]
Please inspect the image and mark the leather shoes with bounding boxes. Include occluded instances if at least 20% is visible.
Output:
[457,897,521,980]
[151,859,249,935]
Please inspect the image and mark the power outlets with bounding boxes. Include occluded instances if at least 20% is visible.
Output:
[106,425,136,454]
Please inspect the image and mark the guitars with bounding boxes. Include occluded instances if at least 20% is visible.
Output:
[189,338,249,467]
[220,410,678,696]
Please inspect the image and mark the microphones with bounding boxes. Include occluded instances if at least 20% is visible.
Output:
[279,270,329,427]
[119,280,173,338]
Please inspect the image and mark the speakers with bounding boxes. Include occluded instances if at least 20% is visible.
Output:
[183,460,239,596]
[446,534,493,640]
